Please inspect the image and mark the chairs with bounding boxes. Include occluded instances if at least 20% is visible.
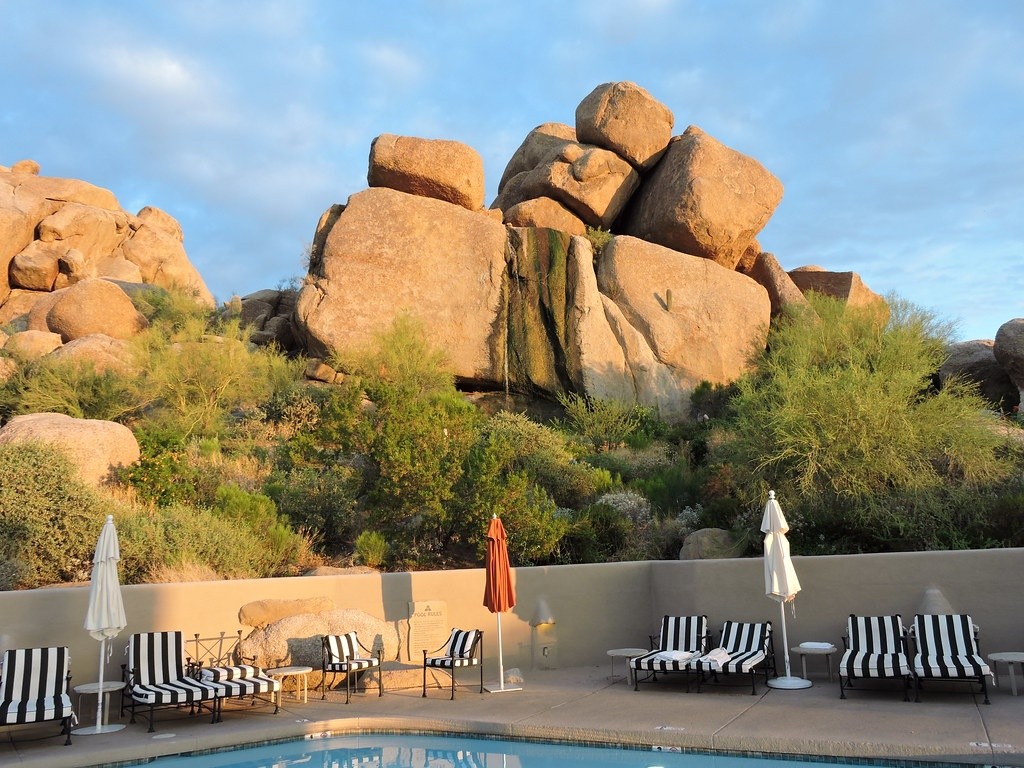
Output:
[696,621,777,695]
[315,630,384,705]
[909,613,997,706]
[121,631,219,733]
[422,627,485,700]
[0,646,73,746]
[327,747,384,768]
[630,615,711,694]
[838,614,915,701]
[424,749,485,768]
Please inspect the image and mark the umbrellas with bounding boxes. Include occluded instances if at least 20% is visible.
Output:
[482,514,522,693]
[760,490,814,689]
[70,514,127,735]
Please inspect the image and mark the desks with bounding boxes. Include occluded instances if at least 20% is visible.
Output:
[70,681,128,736]
[607,648,650,686]
[791,647,838,684]
[267,667,313,708]
[388,747,415,768]
[987,651,1024,697]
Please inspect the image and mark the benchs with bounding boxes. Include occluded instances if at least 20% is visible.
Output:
[194,629,281,723]
